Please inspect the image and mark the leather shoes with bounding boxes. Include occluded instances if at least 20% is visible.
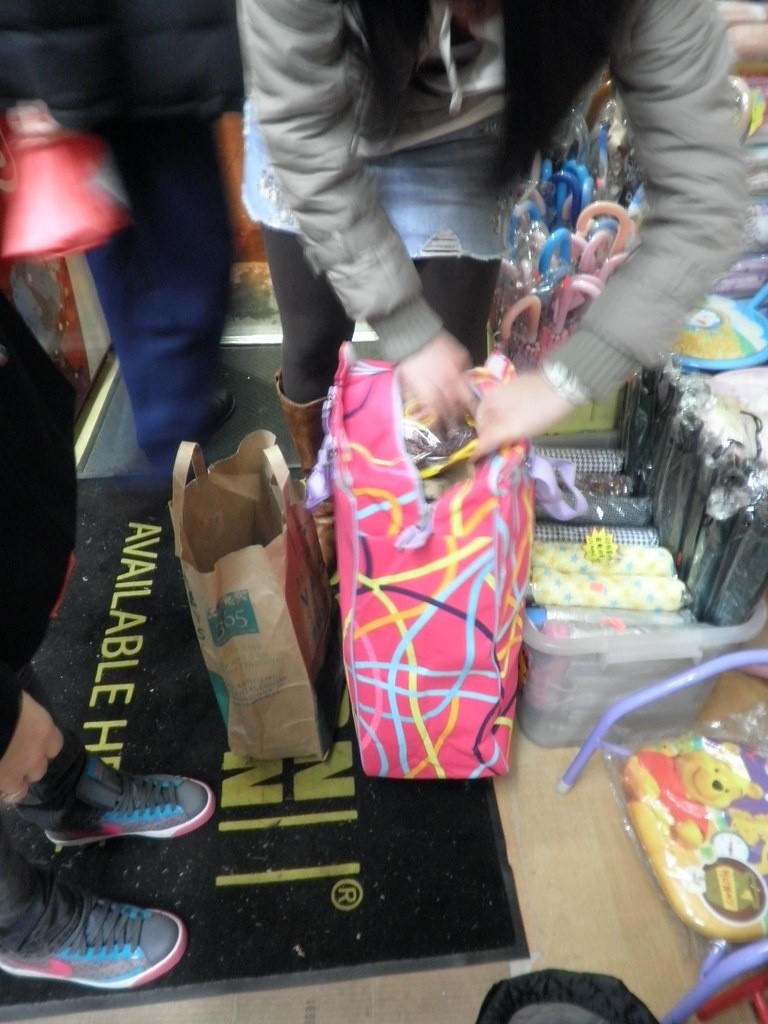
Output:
[148,388,236,463]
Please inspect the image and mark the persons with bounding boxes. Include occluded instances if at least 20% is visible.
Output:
[0,294,217,990]
[0,0,247,481]
[237,0,747,580]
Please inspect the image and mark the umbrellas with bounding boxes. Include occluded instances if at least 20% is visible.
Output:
[494,71,767,709]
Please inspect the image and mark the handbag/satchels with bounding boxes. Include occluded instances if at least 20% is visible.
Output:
[331,355,535,776]
[166,427,339,764]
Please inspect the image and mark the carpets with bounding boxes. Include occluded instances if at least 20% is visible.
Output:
[0,463,533,1024]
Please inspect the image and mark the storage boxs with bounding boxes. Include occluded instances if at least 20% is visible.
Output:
[536,385,621,432]
[533,432,622,449]
[524,600,768,749]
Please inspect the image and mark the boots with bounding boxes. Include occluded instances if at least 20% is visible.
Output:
[275,369,334,569]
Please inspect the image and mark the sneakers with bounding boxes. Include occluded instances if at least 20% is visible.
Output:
[0,897,187,989]
[45,752,216,846]
